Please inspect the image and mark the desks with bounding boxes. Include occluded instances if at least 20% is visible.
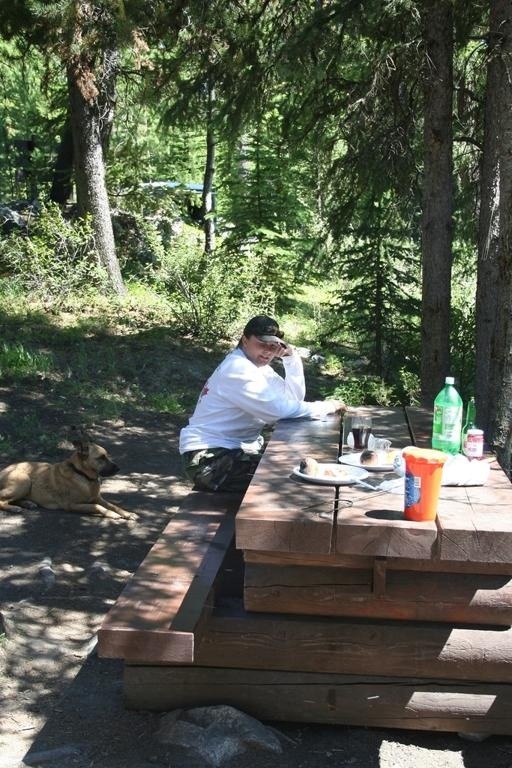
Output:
[235,409,512,628]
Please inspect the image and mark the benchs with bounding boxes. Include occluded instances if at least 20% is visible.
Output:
[98,485,244,707]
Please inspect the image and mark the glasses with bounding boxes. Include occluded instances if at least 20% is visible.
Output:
[257,325,284,338]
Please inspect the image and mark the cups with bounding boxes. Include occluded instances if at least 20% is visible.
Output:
[351,414,372,453]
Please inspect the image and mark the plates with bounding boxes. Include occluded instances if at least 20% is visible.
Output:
[292,462,371,486]
[338,447,402,471]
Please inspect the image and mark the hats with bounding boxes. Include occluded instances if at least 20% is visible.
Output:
[244,315,287,348]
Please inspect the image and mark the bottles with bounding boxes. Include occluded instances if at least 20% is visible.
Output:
[342,409,372,455]
[402,446,448,521]
[431,376,464,457]
[461,396,478,454]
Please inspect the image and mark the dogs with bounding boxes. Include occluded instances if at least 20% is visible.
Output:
[0,433,141,521]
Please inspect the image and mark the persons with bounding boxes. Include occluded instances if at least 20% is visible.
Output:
[178,315,348,493]
